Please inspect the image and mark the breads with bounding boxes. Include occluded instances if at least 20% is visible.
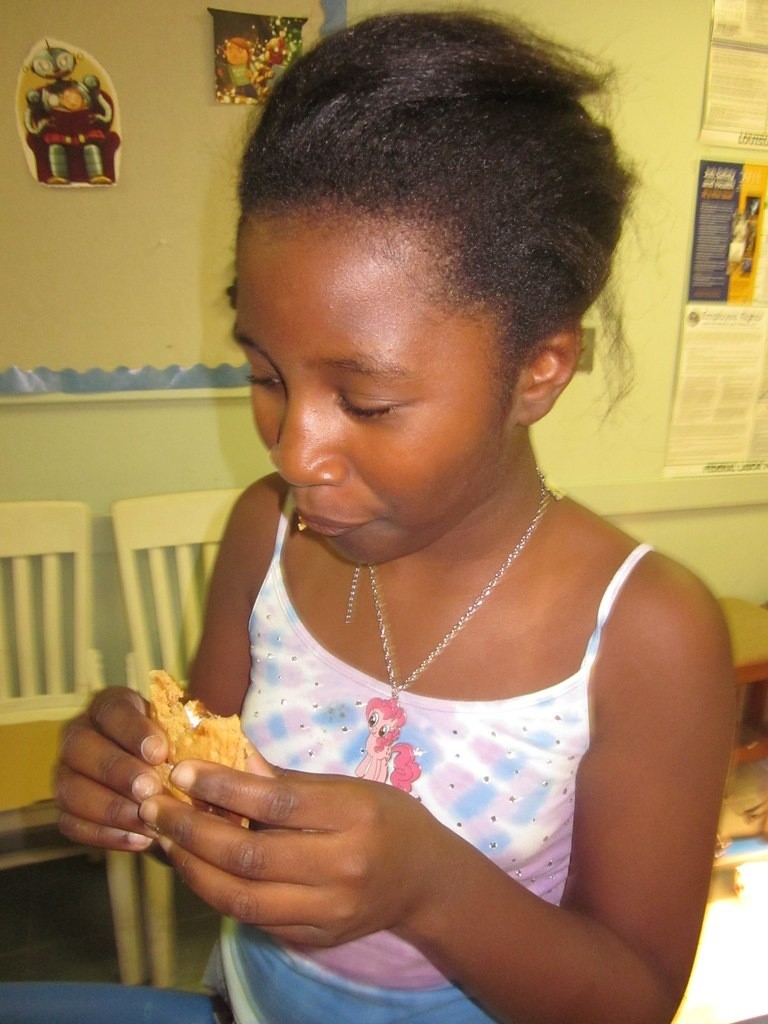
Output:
[146,667,251,831]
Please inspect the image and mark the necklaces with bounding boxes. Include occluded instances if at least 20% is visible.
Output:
[344,468,551,794]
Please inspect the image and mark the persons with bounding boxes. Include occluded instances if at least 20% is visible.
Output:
[49,9,740,1024]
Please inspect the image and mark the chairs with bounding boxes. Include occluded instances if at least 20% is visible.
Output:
[111,477,250,990]
[0,503,144,985]
